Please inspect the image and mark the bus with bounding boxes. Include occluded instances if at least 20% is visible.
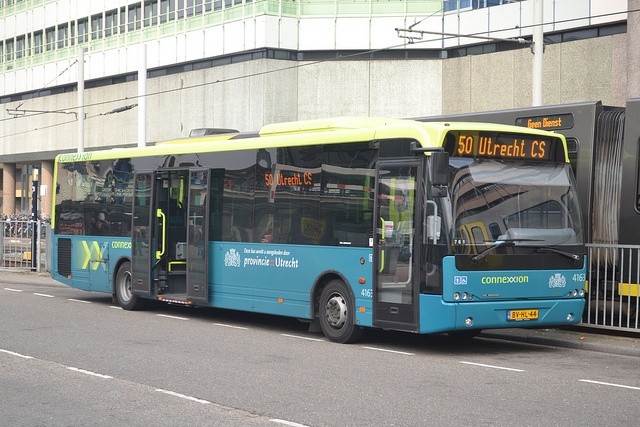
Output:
[47,117,587,341]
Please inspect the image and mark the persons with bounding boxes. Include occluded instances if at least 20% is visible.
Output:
[90,218,106,233]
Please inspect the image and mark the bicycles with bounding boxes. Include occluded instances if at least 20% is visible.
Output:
[2,213,51,237]
